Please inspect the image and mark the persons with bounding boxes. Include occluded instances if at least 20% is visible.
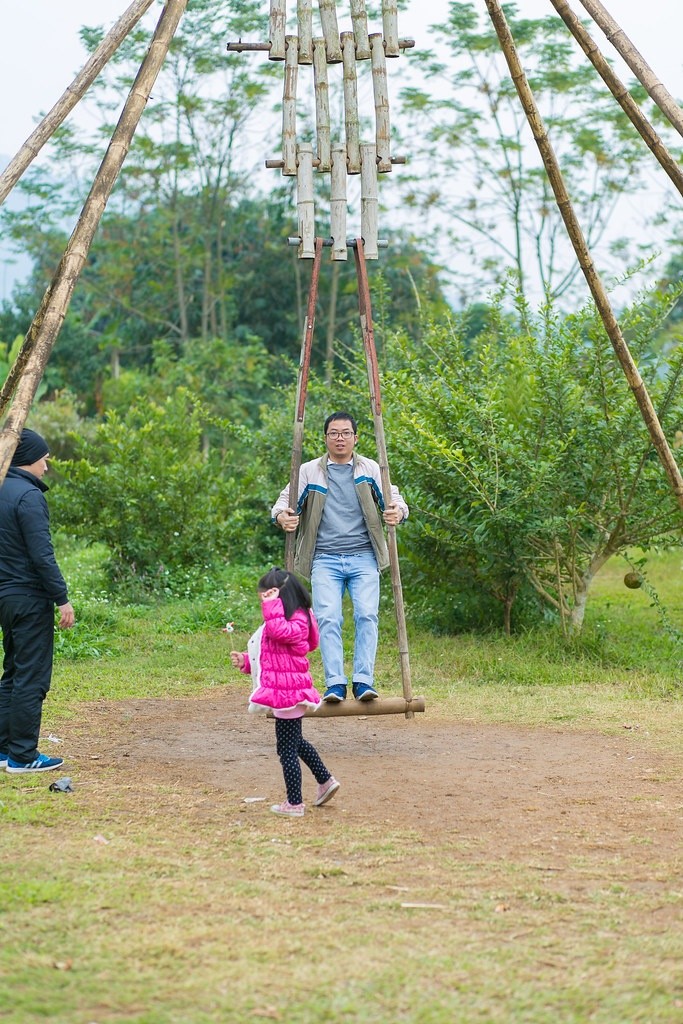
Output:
[271,412,409,700]
[230,568,339,816]
[0,428,75,773]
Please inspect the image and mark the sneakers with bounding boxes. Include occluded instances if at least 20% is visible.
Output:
[323,684,347,702]
[0,752,65,773]
[352,682,379,701]
[269,799,306,817]
[315,775,341,805]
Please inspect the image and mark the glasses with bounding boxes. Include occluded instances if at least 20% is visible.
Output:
[325,431,356,440]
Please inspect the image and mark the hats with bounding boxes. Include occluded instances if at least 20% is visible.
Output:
[10,427,51,467]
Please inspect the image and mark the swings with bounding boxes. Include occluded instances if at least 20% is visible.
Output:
[262,235,426,722]
